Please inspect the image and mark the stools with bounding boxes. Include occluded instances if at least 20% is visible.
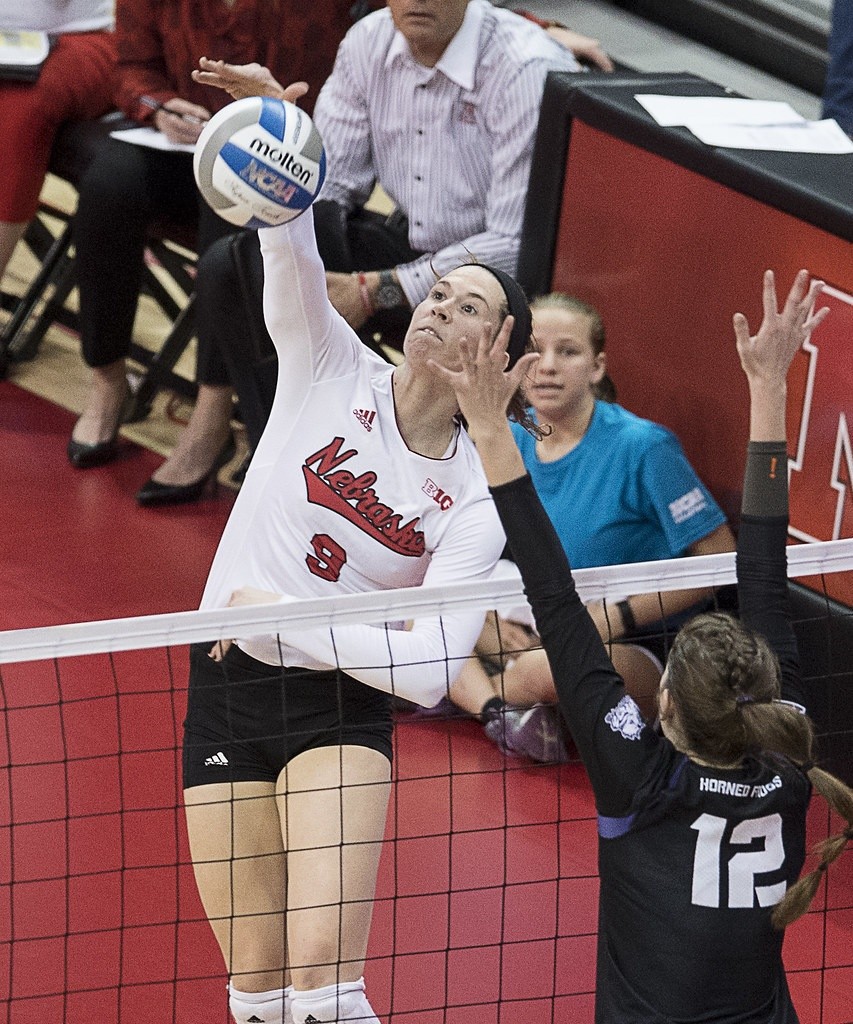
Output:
[0,140,395,483]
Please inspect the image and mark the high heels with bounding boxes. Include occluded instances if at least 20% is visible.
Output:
[136,426,237,506]
[67,384,132,467]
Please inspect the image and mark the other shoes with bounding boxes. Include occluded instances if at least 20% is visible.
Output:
[484,701,571,761]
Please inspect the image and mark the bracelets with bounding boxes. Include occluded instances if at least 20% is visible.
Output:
[615,600,635,635]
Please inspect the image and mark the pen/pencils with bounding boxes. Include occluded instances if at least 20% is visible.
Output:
[134,94,203,124]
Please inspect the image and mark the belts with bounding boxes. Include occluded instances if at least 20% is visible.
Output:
[384,209,409,238]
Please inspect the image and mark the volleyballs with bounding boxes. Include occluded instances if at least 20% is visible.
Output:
[193,96,326,228]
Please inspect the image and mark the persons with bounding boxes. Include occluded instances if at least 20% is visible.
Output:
[0,1,738,766]
[181,57,553,1024]
[428,267,853,1021]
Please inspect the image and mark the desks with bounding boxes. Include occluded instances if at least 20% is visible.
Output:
[514,69,853,787]
